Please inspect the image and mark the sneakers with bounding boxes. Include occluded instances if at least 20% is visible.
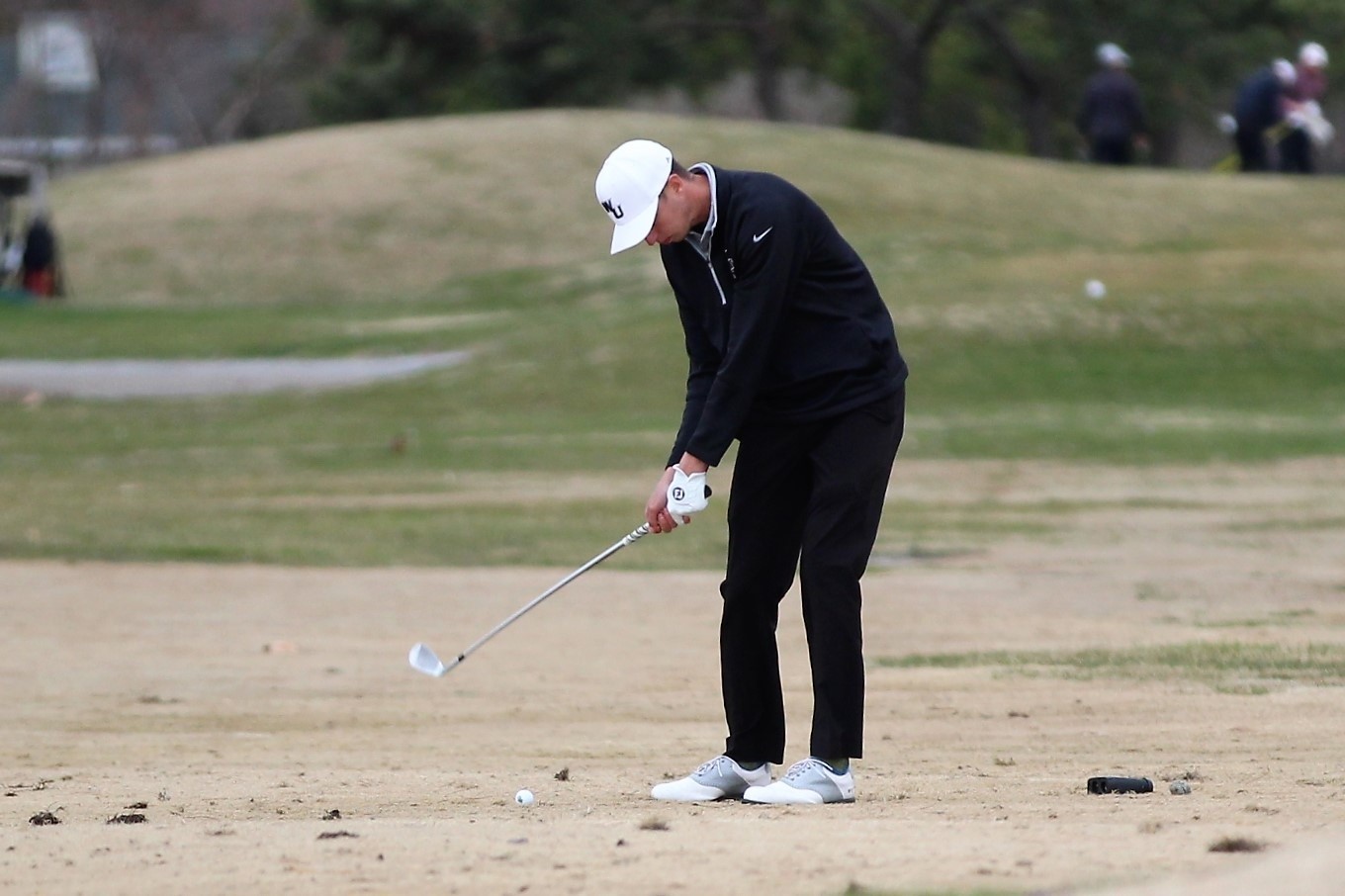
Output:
[650,754,771,802]
[744,757,855,804]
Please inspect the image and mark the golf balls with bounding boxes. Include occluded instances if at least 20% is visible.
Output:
[1087,281,1107,296]
[515,790,534,804]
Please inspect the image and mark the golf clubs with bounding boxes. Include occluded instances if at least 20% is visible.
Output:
[408,484,712,678]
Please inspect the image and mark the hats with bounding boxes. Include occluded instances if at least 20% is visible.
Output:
[596,139,673,253]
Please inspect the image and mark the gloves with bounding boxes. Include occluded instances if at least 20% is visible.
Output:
[667,468,708,526]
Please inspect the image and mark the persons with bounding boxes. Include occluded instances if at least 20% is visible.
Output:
[1075,41,1140,168]
[595,139,910,807]
[1229,40,1335,173]
